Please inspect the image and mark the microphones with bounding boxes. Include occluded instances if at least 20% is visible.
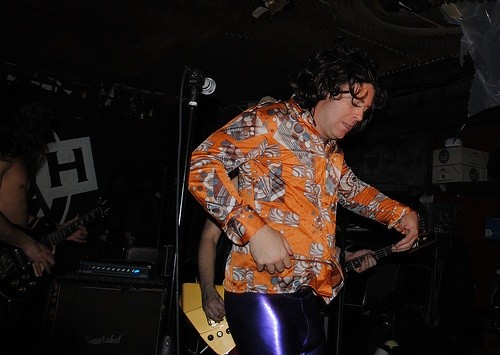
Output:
[185,66,216,95]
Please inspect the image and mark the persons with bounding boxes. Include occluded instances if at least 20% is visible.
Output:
[0,100,89,304]
[187,47,426,355]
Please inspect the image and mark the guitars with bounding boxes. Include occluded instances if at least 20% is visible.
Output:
[0,196,112,301]
[179,229,437,355]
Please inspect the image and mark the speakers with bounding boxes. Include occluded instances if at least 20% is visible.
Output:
[42,280,166,355]
